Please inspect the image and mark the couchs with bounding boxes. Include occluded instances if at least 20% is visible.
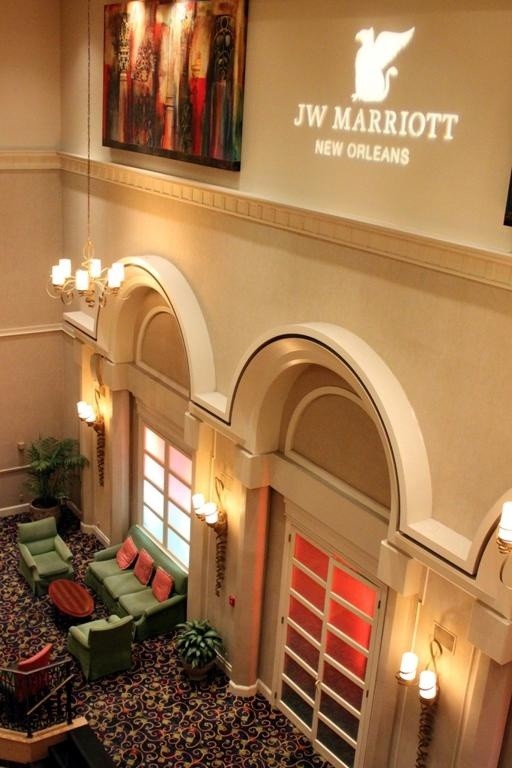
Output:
[86,526,187,641]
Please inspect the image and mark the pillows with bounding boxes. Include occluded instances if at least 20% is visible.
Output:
[115,536,138,570]
[132,548,154,585]
[152,566,174,603]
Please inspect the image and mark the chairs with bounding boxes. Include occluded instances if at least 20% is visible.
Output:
[0,643,54,726]
[67,613,133,682]
[16,516,75,596]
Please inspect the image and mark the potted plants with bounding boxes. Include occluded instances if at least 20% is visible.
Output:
[23,431,89,523]
[174,619,224,681]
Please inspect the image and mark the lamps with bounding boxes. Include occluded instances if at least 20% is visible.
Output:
[192,491,227,595]
[46,0,124,310]
[394,641,442,768]
[495,502,512,591]
[77,401,104,488]
